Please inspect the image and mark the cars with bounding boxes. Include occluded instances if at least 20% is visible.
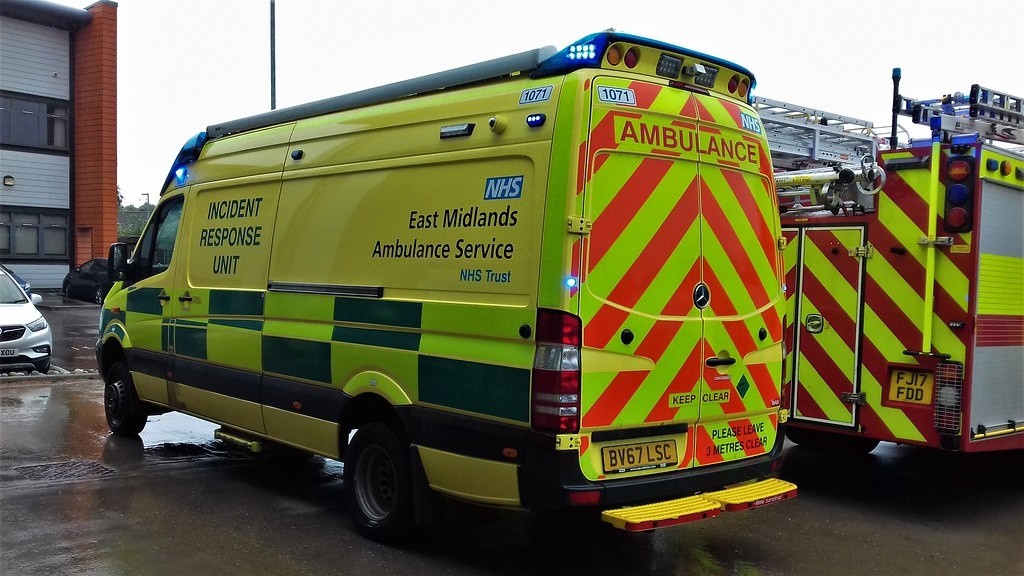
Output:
[62,258,115,304]
[0,263,32,298]
[0,269,52,373]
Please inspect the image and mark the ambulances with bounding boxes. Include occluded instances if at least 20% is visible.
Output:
[96,30,797,556]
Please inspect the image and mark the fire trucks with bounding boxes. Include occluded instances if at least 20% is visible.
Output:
[748,66,1024,453]
